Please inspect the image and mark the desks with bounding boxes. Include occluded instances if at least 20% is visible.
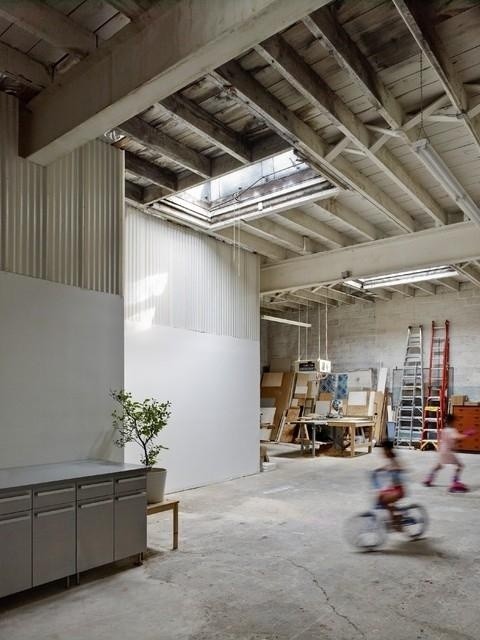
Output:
[284,419,378,458]
[145,499,181,550]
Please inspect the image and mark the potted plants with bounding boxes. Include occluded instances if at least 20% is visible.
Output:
[105,387,173,504]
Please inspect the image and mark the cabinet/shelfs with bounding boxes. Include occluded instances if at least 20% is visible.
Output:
[451,400,480,453]
[0,458,152,601]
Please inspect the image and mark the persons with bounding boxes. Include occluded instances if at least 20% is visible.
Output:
[421,413,477,493]
[371,440,405,533]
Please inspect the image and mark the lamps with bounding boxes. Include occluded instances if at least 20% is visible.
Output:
[261,296,313,328]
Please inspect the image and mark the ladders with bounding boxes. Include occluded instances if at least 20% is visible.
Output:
[392,320,448,451]
